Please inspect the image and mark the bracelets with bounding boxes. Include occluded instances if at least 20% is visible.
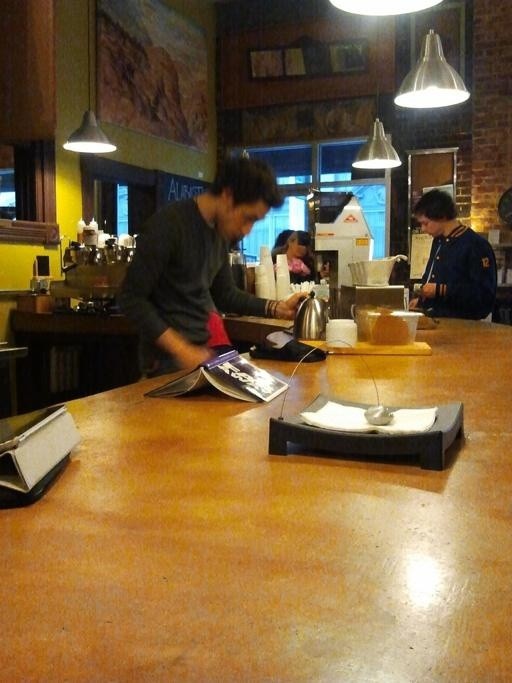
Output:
[435,281,441,297]
[264,298,283,319]
[441,282,445,297]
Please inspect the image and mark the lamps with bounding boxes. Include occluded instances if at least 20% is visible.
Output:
[326,0,469,168]
[62,0,119,157]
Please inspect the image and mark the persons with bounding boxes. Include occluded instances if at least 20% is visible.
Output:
[110,153,312,390]
[267,229,317,286]
[407,186,498,323]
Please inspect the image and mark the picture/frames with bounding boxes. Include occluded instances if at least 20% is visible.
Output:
[86,0,213,153]
[244,37,369,80]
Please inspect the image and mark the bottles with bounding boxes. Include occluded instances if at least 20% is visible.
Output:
[77,216,99,247]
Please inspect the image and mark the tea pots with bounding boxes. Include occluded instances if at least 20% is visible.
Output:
[288,289,333,342]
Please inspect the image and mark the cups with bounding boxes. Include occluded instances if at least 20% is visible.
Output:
[252,244,290,301]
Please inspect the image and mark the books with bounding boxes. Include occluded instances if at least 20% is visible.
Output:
[142,348,290,406]
